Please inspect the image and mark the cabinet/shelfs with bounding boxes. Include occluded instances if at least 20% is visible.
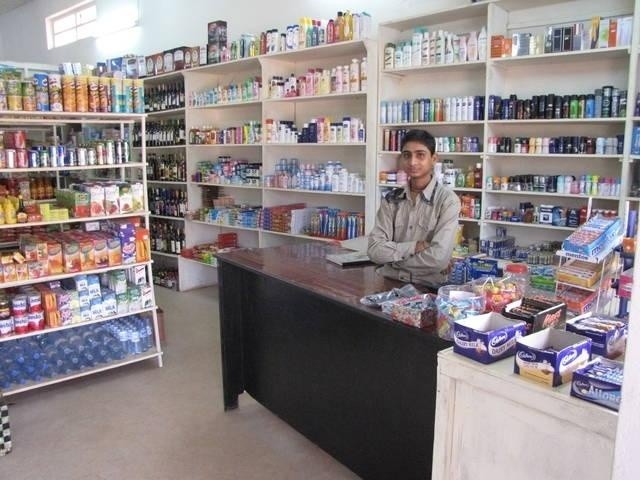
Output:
[0,59,169,397]
[431,347,616,478]
[378,0,639,300]
[181,38,372,294]
[130,68,188,292]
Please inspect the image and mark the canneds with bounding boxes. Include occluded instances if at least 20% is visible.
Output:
[0,130,130,168]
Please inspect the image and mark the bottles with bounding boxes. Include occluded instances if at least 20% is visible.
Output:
[0,315,153,396]
[143,81,188,292]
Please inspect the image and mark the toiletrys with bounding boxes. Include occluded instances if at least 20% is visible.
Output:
[0,285,45,338]
[378,85,627,229]
[189,9,368,195]
[379,95,637,238]
[384,24,486,64]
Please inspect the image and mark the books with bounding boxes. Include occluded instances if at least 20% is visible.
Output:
[325,249,375,266]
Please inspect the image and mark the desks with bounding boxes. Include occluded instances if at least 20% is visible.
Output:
[208,234,525,478]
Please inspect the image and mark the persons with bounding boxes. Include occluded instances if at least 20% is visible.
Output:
[365,128,463,293]
